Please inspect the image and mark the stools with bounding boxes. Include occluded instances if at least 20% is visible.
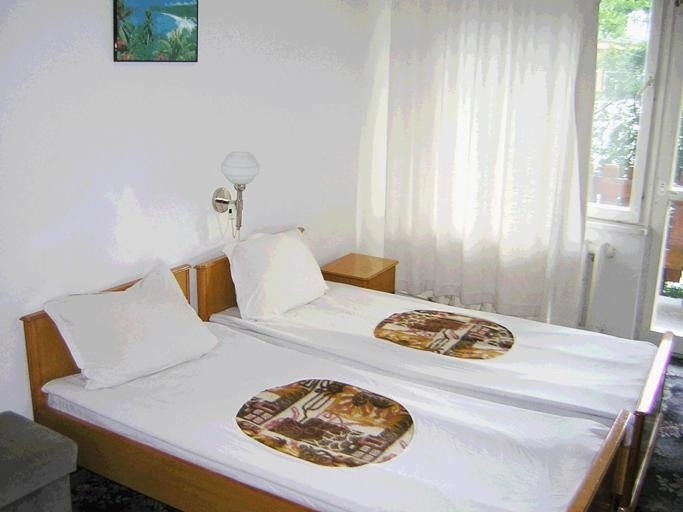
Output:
[1,408,76,512]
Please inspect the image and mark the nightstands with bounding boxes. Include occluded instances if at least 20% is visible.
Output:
[320,251,397,293]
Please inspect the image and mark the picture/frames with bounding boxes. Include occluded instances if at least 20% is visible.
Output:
[111,0,199,66]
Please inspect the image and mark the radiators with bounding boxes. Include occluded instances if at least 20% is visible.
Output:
[425,236,614,334]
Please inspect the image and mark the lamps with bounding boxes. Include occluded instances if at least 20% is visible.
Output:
[211,149,261,238]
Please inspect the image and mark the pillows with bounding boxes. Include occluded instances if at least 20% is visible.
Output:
[44,258,216,390]
[222,227,329,323]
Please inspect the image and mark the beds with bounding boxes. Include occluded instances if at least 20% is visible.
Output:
[194,226,676,512]
[22,263,636,511]
